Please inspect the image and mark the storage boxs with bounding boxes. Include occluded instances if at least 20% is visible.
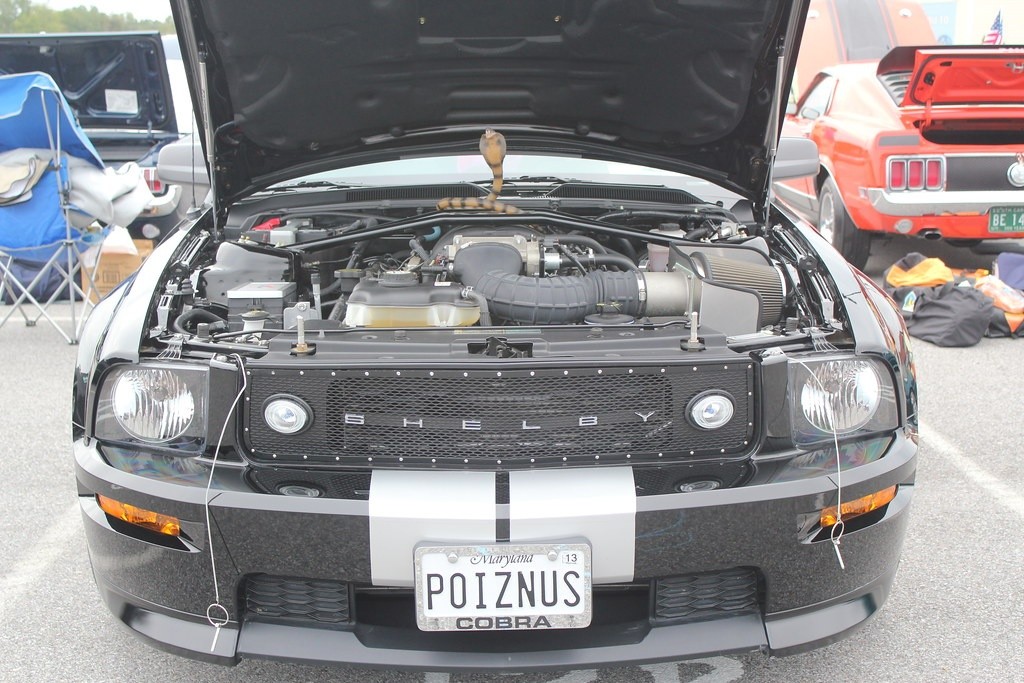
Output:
[881,250,1024,348]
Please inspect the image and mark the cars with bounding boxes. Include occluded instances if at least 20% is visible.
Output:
[70,1,918,674]
[769,0,1023,274]
[1,29,195,239]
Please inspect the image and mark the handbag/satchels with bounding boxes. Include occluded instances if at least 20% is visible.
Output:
[884,250,1023,344]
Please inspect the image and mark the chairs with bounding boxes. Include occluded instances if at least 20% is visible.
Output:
[0,69,152,346]
